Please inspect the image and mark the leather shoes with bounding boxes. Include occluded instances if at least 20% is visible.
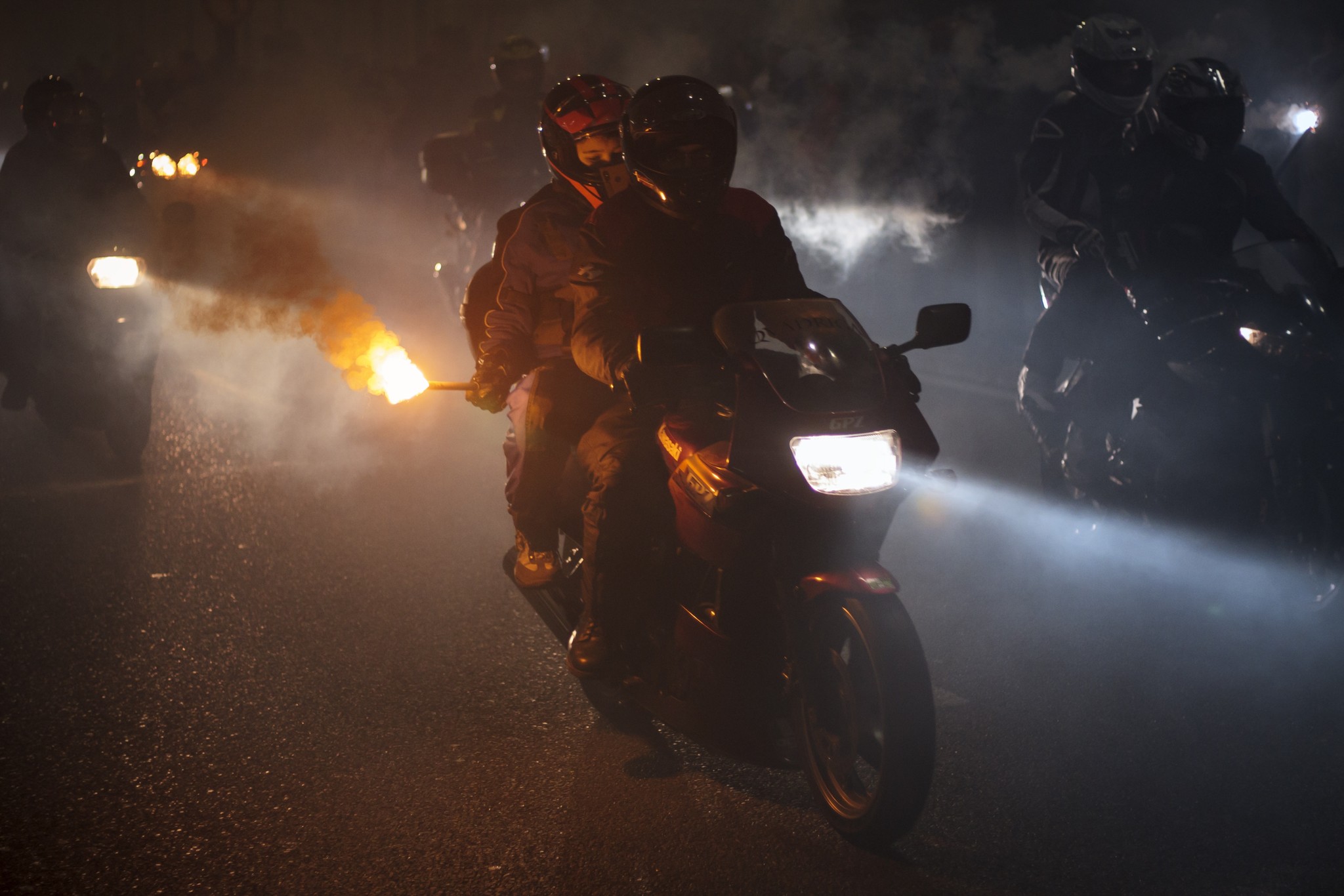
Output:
[565,612,619,680]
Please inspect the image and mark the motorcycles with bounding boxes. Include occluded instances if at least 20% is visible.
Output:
[501,302,970,850]
[1025,265,1344,562]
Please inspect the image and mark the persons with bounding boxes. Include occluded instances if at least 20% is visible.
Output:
[566,75,922,683]
[1023,58,1344,516]
[472,34,552,190]
[1020,12,1155,292]
[460,72,631,584]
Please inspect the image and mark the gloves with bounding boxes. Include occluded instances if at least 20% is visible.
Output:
[876,348,923,397]
[463,368,510,414]
[617,353,679,412]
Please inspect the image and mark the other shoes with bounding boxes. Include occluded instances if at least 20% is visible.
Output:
[1062,422,1108,488]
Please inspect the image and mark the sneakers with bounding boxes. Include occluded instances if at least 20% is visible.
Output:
[513,528,558,590]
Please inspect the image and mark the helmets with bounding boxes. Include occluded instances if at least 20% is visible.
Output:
[536,76,638,209]
[490,35,551,99]
[1070,16,1154,115]
[616,74,739,215]
[1147,56,1246,160]
